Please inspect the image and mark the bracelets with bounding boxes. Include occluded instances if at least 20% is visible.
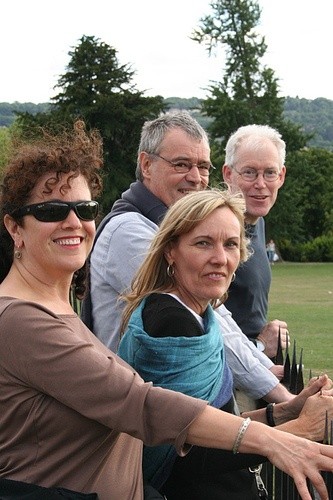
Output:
[266,403,276,427]
[232,417,252,455]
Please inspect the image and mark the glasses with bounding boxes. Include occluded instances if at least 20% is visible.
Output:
[10,200,99,222]
[230,165,284,179]
[152,152,216,176]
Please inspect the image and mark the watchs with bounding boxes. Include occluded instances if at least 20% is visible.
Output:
[254,338,265,352]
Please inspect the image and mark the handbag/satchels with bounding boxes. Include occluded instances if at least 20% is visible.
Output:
[180,442,267,500]
[0,478,98,500]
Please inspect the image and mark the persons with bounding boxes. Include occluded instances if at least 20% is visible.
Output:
[80,109,306,404]
[0,120,333,500]
[266,239,275,264]
[221,123,306,413]
[114,182,333,500]
